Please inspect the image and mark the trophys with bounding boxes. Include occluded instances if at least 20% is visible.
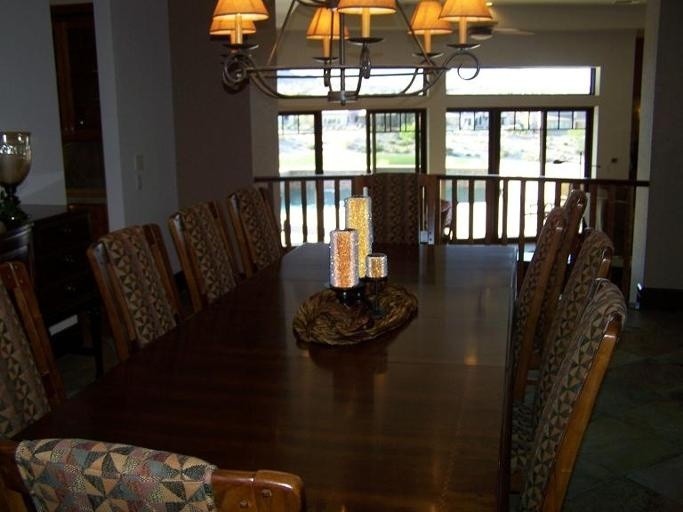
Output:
[0,128,34,221]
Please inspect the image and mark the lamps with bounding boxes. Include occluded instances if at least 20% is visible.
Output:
[345,196,372,294]
[329,228,358,311]
[210,1,500,105]
[366,253,387,301]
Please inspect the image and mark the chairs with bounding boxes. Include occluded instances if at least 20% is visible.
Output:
[4,439,303,509]
[170,200,241,308]
[531,189,588,368]
[88,225,185,365]
[354,173,436,248]
[504,280,625,508]
[511,208,564,411]
[510,225,614,481]
[4,260,63,436]
[220,182,285,277]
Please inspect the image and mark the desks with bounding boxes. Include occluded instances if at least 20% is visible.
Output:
[3,241,517,508]
